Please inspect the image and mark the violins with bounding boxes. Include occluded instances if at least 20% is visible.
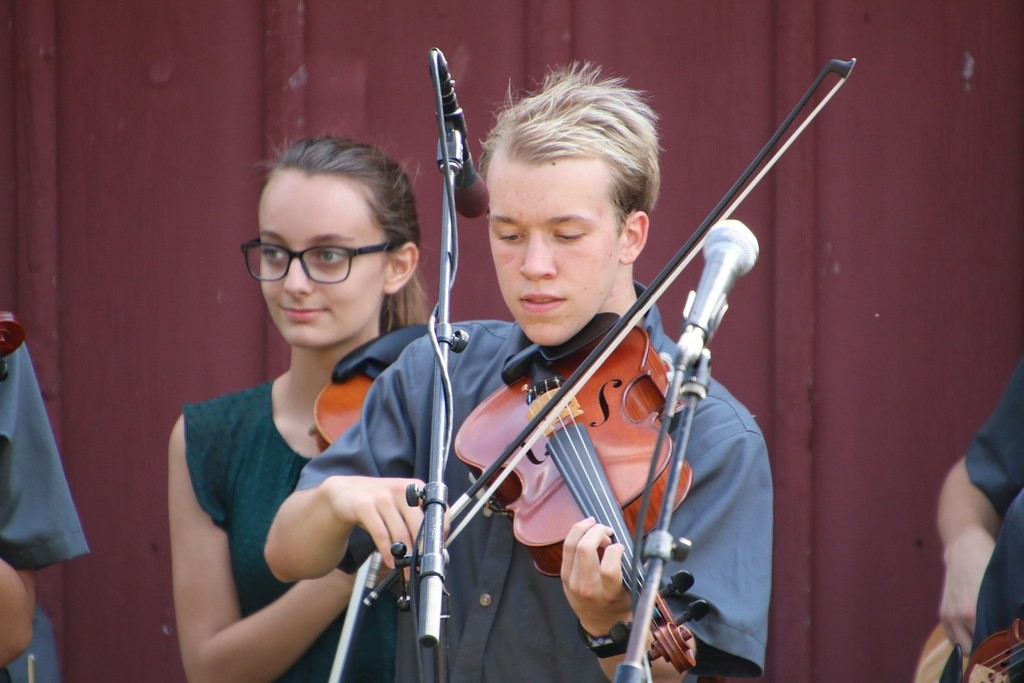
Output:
[0,305,25,384]
[312,311,710,676]
[962,617,1024,683]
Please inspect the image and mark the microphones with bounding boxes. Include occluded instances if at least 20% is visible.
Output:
[676,218,760,374]
[430,47,490,219]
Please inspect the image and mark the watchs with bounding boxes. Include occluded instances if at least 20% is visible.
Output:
[576,619,626,659]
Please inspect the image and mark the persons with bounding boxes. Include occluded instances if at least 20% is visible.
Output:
[936,355,1024,683]
[167,133,424,683]
[0,341,85,683]
[262,75,773,682]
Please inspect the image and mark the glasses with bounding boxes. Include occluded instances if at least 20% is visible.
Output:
[241,238,401,285]
